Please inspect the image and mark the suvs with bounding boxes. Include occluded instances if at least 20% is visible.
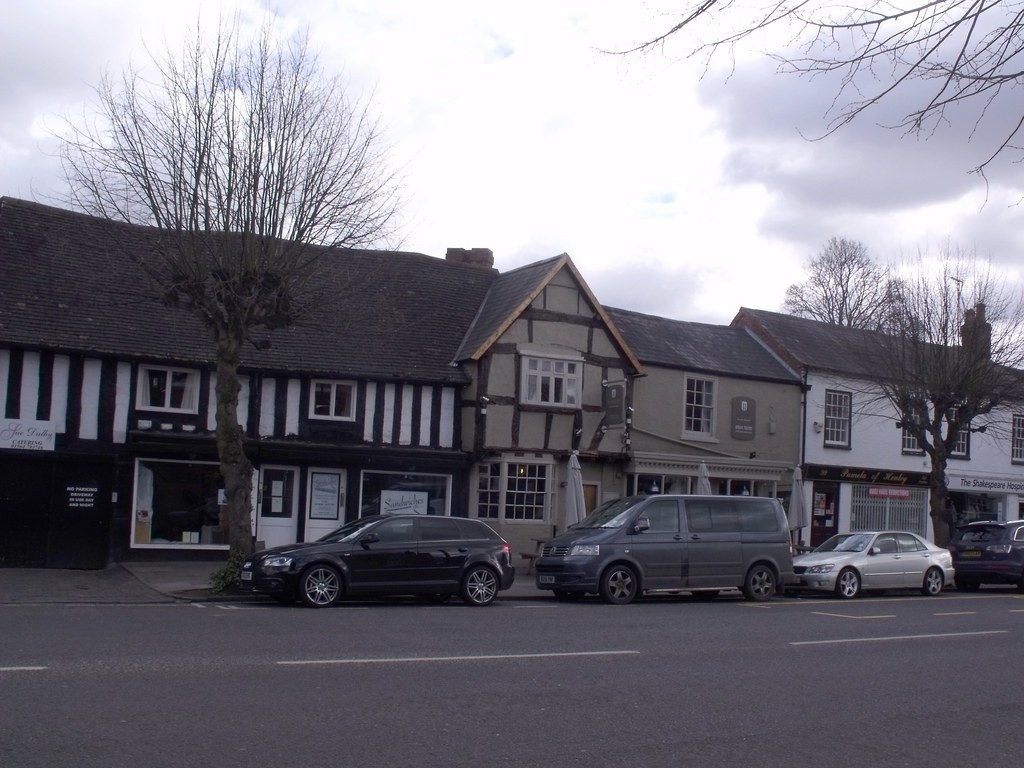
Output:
[946,519,1024,592]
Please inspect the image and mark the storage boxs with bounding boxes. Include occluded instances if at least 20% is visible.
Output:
[181,531,200,544]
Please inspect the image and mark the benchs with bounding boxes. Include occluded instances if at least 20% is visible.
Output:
[519,551,539,575]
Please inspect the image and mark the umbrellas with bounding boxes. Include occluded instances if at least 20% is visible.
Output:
[788,464,809,555]
[696,460,711,495]
[564,451,586,532]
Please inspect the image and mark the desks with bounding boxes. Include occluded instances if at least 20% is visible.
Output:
[530,538,553,553]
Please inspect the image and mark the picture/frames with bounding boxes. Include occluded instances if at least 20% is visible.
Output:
[309,472,341,520]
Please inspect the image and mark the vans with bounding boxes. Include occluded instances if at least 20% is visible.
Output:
[534,494,797,605]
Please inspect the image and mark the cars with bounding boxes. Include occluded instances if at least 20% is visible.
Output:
[790,531,956,599]
[238,514,516,610]
